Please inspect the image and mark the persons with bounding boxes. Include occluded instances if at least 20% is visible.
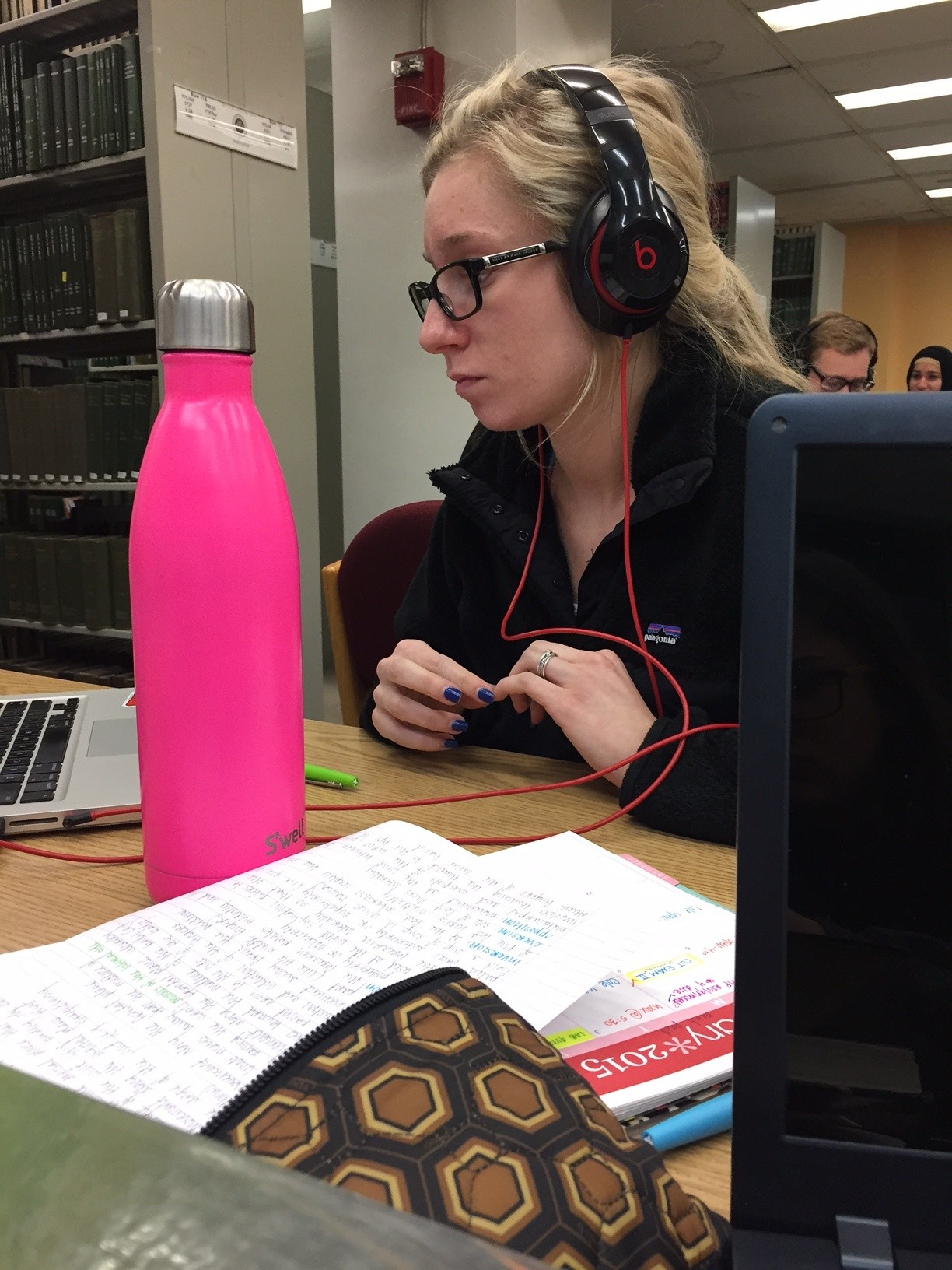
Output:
[797,311,875,392]
[905,345,952,393]
[364,61,817,845]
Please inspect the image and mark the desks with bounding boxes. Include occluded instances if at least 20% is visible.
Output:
[0,664,731,1221]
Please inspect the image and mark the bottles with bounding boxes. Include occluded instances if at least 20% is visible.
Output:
[125,278,310,899]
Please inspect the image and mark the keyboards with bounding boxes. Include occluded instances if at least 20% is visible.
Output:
[0,688,142,837]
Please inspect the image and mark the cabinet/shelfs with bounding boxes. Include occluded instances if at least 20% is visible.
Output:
[2,0,847,685]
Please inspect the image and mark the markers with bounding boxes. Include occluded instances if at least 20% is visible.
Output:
[642,1089,734,1151]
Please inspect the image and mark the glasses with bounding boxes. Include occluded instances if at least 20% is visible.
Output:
[807,361,876,392]
[407,239,559,323]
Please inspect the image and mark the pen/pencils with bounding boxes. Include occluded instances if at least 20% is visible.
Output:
[304,764,359,789]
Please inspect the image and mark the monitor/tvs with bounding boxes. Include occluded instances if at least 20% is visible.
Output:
[729,391,952,1270]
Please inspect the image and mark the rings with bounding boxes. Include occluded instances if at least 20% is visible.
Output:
[536,648,559,679]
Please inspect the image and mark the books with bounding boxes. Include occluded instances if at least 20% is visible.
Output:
[0,0,158,636]
[704,181,730,254]
[480,830,736,1157]
[770,233,814,363]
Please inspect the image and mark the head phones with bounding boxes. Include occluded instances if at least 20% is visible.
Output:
[517,62,691,339]
[788,315,879,391]
